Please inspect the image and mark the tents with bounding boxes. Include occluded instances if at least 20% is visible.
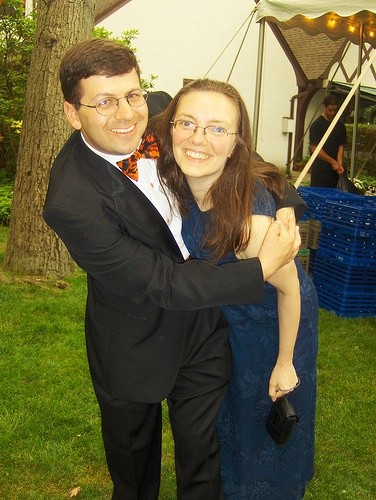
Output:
[250,0,376,149]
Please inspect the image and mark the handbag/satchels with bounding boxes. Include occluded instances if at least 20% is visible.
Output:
[266,397,298,449]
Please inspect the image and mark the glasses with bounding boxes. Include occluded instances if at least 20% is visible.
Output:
[70,88,149,116]
[169,120,238,138]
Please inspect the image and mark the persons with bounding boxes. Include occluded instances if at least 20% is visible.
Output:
[309,93,347,188]
[144,78,319,500]
[41,37,309,500]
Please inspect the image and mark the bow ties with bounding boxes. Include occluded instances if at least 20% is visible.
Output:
[116,134,159,182]
